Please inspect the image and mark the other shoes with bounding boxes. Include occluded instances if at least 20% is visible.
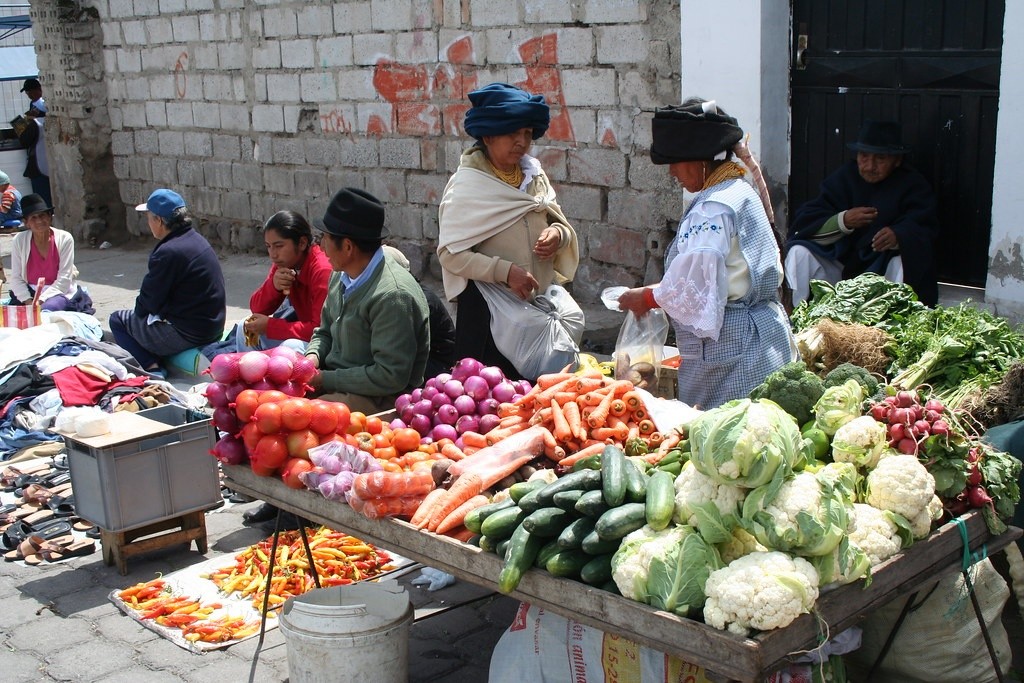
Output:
[147,367,166,380]
[244,504,313,532]
[220,485,256,502]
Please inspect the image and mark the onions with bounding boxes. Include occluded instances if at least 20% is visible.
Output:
[389,358,532,450]
[205,345,315,464]
[300,441,387,500]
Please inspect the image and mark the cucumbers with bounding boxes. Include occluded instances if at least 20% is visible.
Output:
[463,444,676,594]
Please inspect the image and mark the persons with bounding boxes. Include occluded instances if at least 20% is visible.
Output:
[8,194,78,314]
[20,78,55,216]
[784,120,928,327]
[0,171,22,227]
[436,81,580,383]
[108,188,226,380]
[380,243,455,391]
[619,98,802,414]
[233,211,335,361]
[243,187,431,534]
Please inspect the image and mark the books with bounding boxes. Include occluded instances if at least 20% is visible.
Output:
[11,115,28,137]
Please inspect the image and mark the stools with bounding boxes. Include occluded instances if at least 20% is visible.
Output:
[95,501,226,578]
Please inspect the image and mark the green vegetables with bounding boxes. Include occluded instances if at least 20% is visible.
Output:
[788,272,1024,440]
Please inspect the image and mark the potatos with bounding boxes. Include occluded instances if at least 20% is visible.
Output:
[615,352,658,394]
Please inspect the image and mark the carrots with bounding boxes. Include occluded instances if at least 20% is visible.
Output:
[463,363,681,465]
[411,433,565,542]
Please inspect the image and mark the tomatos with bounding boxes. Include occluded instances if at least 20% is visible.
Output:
[281,459,313,489]
[232,391,453,471]
[348,470,436,520]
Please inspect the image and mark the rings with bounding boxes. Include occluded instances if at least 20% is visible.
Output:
[885,240,888,244]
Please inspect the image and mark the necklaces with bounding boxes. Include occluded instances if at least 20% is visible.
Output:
[483,154,524,188]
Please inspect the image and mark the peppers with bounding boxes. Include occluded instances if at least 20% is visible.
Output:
[200,521,398,618]
[573,422,696,481]
[116,572,261,645]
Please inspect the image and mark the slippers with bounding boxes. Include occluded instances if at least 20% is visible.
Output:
[0,431,108,572]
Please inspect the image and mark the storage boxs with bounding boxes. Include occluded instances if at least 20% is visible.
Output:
[64,403,225,532]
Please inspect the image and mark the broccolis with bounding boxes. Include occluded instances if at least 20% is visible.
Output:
[690,360,943,637]
[610,465,747,617]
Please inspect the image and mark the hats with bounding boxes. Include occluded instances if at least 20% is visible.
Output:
[134,188,187,223]
[19,193,56,219]
[464,82,551,140]
[846,116,914,156]
[313,187,388,242]
[18,78,41,92]
[648,95,744,165]
[0,171,11,185]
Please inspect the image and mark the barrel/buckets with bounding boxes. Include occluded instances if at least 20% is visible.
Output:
[278,576,415,683]
